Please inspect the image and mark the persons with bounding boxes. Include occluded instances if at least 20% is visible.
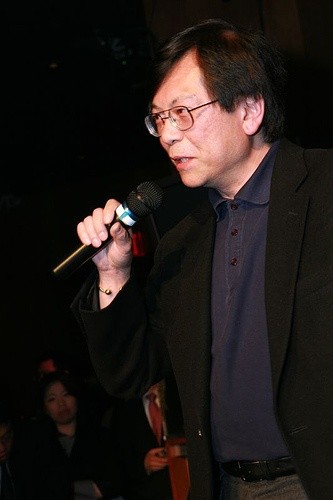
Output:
[73,18,331,500]
[0,375,200,500]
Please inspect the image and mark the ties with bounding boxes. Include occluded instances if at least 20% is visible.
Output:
[144,392,164,449]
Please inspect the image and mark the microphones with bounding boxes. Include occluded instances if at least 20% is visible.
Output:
[51,181,165,282]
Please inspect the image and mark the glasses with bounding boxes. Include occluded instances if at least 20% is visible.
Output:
[144,97,220,138]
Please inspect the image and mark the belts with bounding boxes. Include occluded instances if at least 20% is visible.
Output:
[212,456,299,484]
